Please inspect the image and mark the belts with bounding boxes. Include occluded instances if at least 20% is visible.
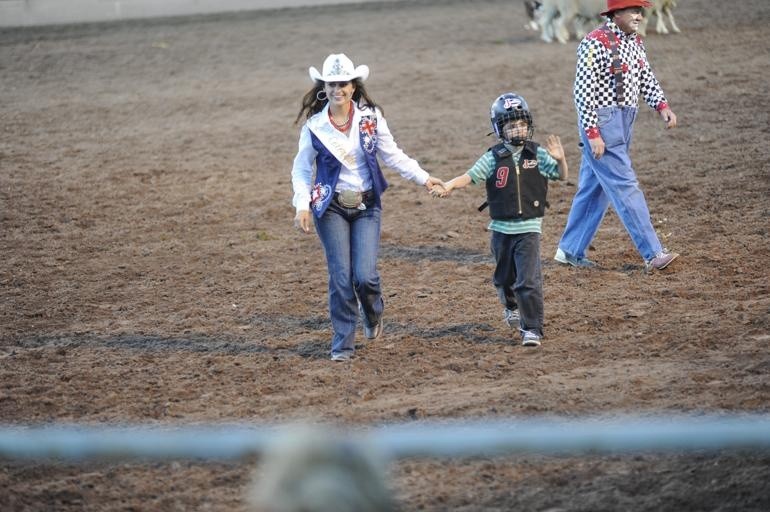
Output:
[333,190,374,201]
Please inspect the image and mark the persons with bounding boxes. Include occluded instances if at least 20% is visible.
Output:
[554,0,680,276]
[290,54,447,361]
[429,92,568,345]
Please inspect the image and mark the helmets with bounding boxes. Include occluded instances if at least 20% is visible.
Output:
[491,93,534,146]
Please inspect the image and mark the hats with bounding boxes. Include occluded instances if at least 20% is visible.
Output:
[309,53,369,83]
[601,0,655,16]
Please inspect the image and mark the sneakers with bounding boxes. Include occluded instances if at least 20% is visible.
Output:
[331,355,351,361]
[554,249,596,268]
[505,309,520,328]
[645,252,680,274]
[365,317,383,339]
[522,331,541,346]
